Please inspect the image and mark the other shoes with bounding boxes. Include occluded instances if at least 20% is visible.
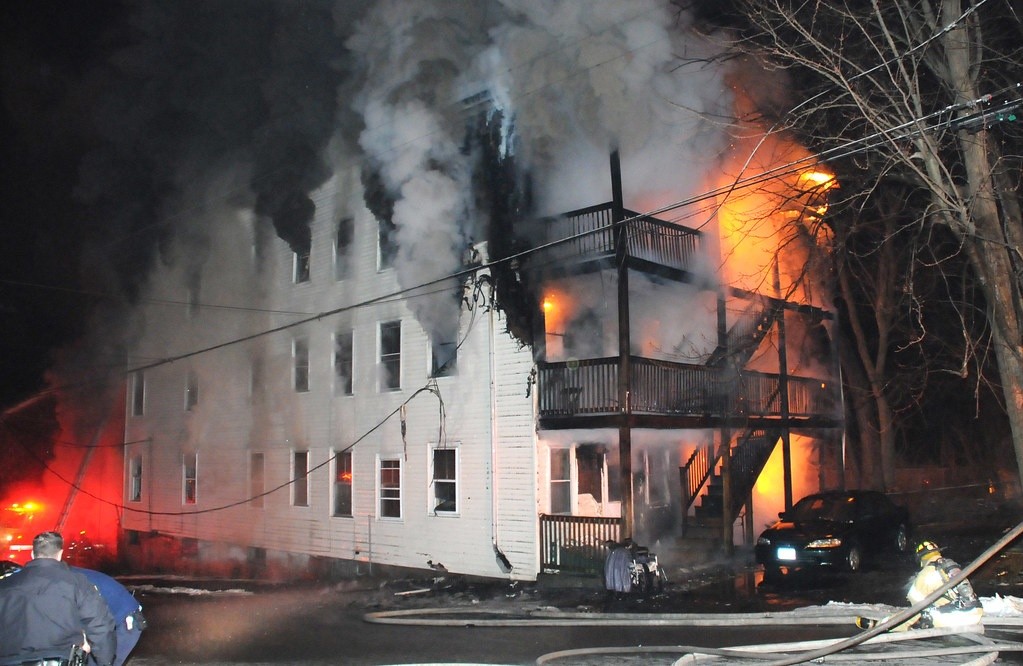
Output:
[855,616,878,629]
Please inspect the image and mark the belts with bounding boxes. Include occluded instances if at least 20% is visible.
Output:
[22,657,68,666]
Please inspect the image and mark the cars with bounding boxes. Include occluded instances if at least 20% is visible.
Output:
[756,487,914,577]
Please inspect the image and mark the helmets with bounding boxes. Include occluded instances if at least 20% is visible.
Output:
[916,541,941,560]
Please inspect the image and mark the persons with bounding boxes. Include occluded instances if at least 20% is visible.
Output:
[67,530,98,565]
[0,561,147,666]
[604,537,633,613]
[0,531,117,666]
[854,540,983,633]
